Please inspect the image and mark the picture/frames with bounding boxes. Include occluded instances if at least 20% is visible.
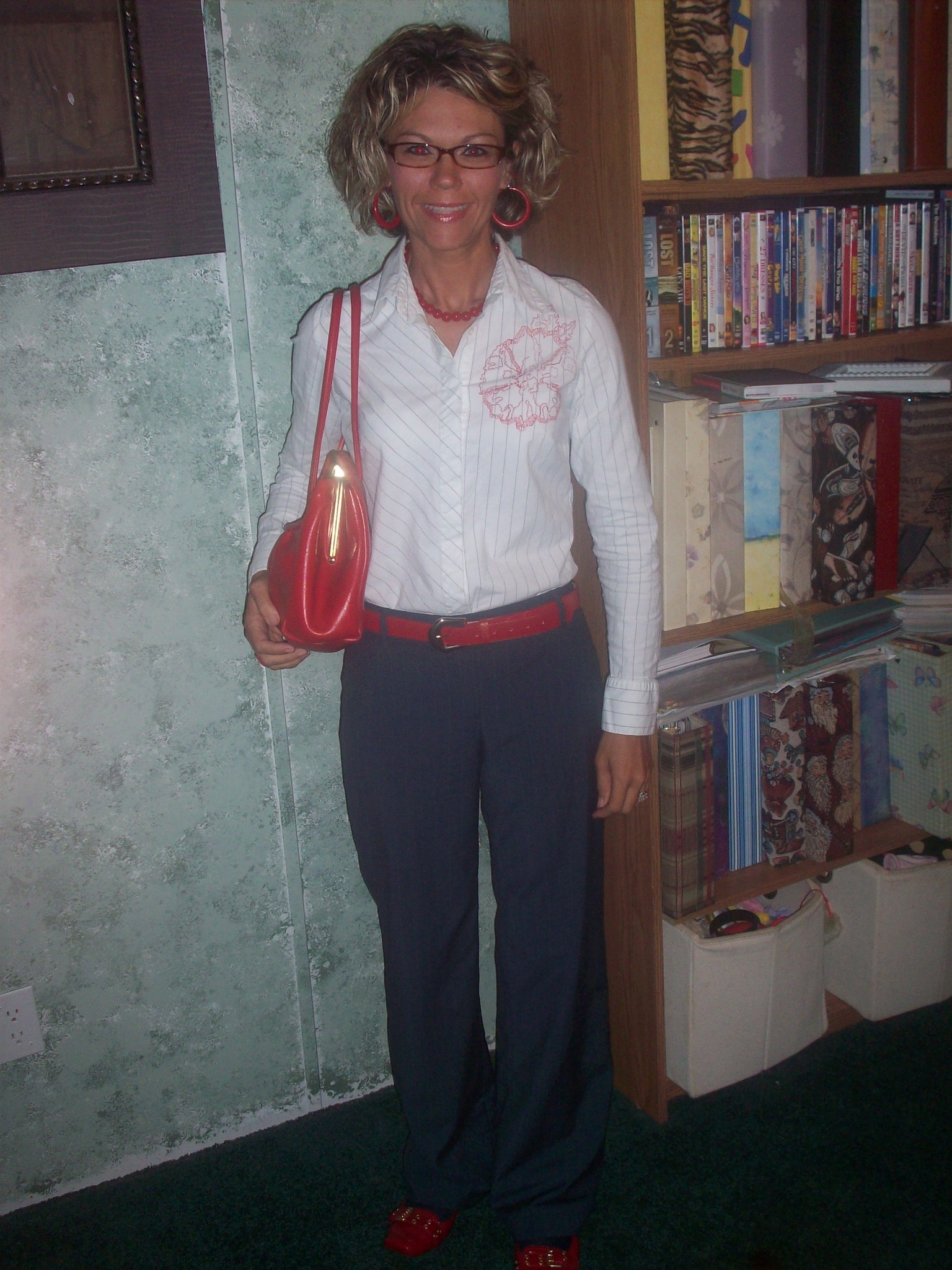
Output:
[0,0,156,196]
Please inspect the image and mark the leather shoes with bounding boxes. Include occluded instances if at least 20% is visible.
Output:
[382,1202,461,1256]
[514,1234,581,1270]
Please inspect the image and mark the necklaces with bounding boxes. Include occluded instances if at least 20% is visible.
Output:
[403,241,499,321]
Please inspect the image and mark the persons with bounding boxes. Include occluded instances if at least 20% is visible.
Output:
[242,23,658,1270]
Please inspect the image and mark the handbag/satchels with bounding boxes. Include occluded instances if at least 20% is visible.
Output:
[267,279,372,652]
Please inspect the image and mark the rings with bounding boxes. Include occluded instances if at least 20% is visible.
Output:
[637,792,648,803]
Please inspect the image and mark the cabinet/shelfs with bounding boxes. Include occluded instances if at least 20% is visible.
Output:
[510,0,952,1125]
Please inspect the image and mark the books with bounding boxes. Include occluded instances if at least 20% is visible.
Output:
[641,187,952,357]
[659,634,952,922]
[647,361,952,720]
[634,0,952,182]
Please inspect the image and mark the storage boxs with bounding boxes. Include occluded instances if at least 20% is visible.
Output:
[821,859,952,1021]
[662,877,828,1100]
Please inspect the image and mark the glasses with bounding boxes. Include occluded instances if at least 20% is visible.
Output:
[381,141,510,169]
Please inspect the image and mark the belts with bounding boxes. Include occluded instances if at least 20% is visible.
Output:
[366,588,580,653]
